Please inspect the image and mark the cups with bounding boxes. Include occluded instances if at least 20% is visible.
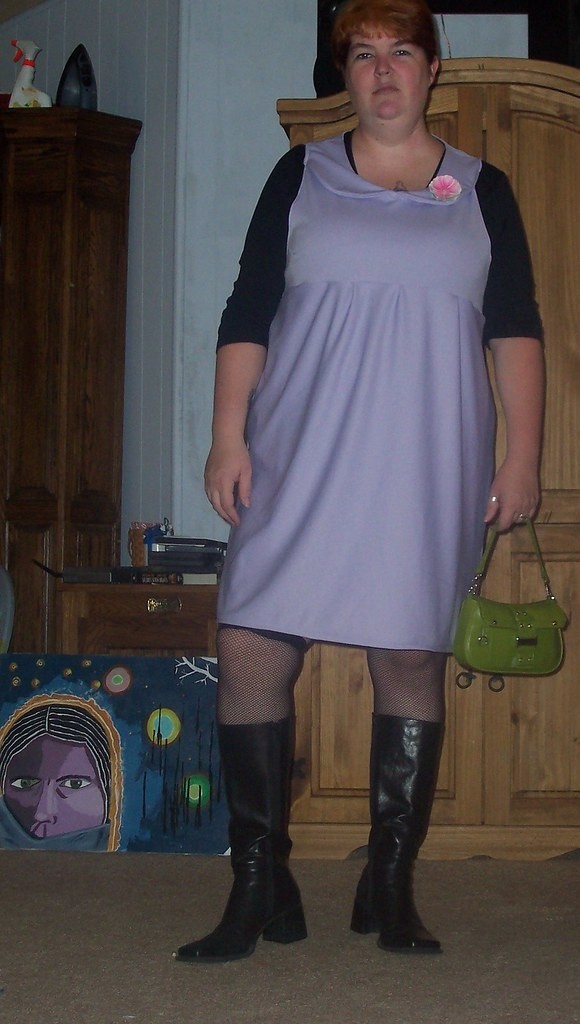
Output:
[128,521,157,567]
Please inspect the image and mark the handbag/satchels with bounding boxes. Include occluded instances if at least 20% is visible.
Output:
[454,515,569,675]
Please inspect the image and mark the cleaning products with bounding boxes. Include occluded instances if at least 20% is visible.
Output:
[8,38,52,108]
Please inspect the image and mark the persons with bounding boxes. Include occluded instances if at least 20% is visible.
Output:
[173,0,547,965]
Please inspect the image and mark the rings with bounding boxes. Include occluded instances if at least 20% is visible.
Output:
[490,496,498,502]
[519,513,528,518]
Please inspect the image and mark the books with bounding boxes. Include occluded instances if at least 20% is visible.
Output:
[152,538,221,587]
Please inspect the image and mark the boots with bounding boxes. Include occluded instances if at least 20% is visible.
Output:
[350,711,444,955]
[174,712,310,964]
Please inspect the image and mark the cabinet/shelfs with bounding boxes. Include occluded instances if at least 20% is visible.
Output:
[0,107,142,655]
[277,57,577,862]
[57,585,217,657]
